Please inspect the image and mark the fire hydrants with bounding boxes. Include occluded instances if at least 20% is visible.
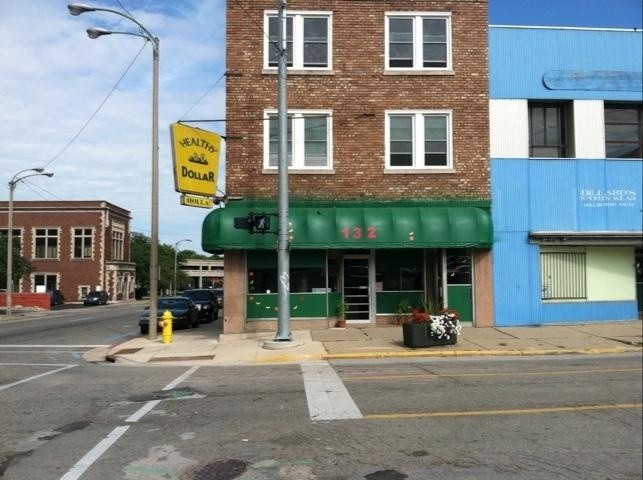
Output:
[159,309,175,344]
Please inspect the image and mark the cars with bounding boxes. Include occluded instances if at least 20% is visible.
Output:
[447,256,471,284]
[83,290,109,305]
[45,290,64,306]
[139,289,223,334]
[0,289,6,293]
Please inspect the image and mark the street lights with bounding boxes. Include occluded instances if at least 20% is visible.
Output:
[68,2,159,341]
[174,240,192,297]
[6,167,54,316]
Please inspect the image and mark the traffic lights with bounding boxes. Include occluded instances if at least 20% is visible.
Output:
[255,216,270,231]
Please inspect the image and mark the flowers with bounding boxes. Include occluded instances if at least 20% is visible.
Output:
[405,292,463,346]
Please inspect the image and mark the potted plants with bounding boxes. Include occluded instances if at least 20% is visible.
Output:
[391,299,409,325]
[334,296,349,328]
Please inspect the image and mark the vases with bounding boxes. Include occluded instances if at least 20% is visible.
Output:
[402,320,460,348]
